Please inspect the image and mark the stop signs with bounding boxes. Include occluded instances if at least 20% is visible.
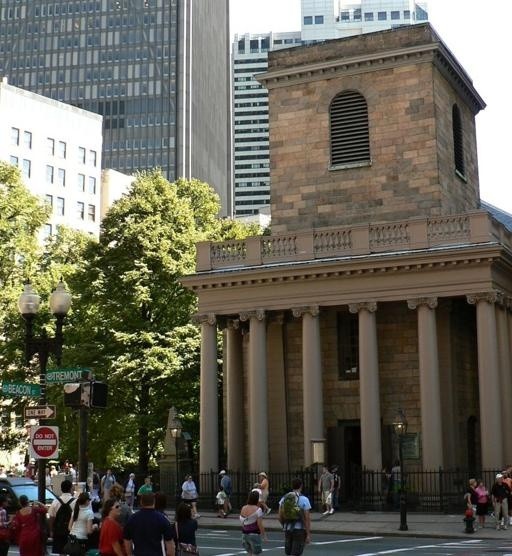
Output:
[28,424,59,458]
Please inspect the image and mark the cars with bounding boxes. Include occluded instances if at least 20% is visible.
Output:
[1,474,63,541]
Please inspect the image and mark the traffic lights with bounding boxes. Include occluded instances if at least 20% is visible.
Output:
[90,380,109,405]
[64,385,80,409]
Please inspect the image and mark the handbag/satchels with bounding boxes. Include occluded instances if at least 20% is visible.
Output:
[64,534,84,556]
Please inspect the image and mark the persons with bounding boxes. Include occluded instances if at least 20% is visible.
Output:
[0,461,201,556]
[219,470,232,513]
[330,466,341,506]
[251,483,262,507]
[216,486,228,518]
[392,459,401,482]
[318,466,335,515]
[259,471,272,514]
[278,478,312,556]
[240,491,264,556]
[464,466,512,531]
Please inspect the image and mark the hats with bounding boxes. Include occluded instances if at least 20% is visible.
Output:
[496,474,503,478]
[259,472,267,478]
[219,470,226,475]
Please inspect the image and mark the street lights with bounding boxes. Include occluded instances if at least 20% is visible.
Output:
[392,407,410,532]
[18,277,71,546]
[170,412,184,496]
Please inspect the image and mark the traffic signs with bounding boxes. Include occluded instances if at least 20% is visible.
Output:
[46,369,86,381]
[23,405,56,420]
[1,381,40,396]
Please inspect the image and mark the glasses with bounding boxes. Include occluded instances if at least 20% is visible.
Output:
[115,506,119,508]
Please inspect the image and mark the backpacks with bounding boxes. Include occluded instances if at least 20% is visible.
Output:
[280,492,304,522]
[53,496,76,540]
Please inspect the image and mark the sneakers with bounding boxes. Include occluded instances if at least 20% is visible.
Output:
[224,512,228,518]
[217,516,222,518]
[496,524,509,530]
[267,508,271,514]
[329,508,334,514]
[323,510,329,514]
[193,513,200,518]
[177,542,199,556]
